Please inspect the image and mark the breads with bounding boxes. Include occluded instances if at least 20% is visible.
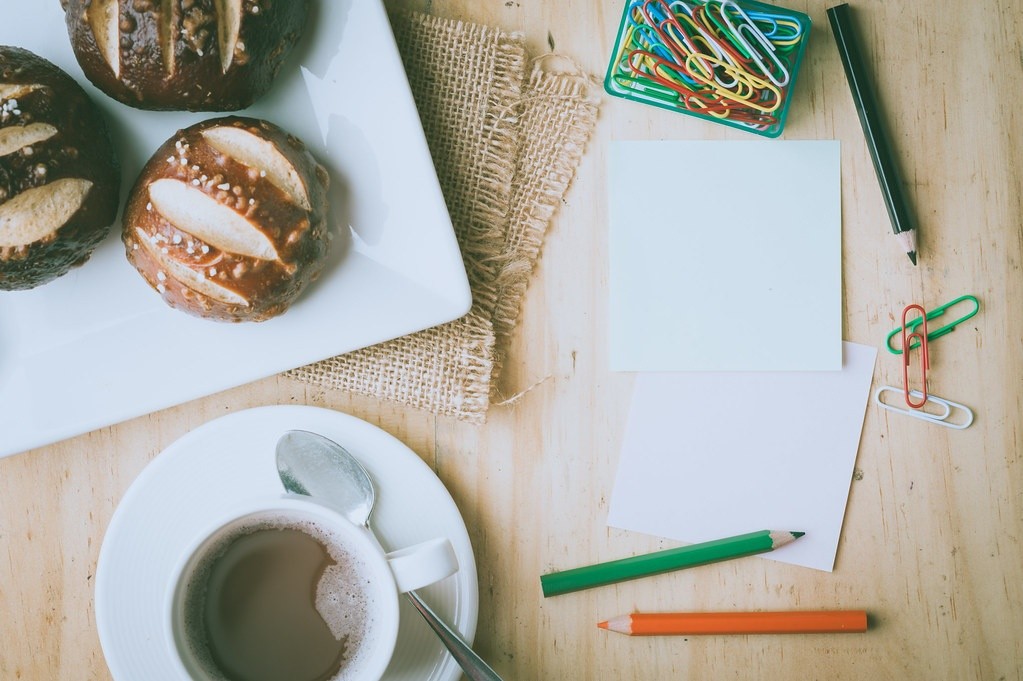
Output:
[0,44,117,292]
[58,0,314,112]
[120,114,330,322]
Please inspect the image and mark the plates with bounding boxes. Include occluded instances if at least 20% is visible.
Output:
[94,406,478,681]
[0,0,473,456]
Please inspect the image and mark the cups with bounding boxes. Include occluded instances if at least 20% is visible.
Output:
[162,493,459,681]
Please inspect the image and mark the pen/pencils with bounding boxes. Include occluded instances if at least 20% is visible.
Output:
[539,530,807,599]
[825,3,920,267]
[596,608,867,637]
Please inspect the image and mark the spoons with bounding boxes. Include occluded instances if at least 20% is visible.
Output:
[274,429,501,681]
